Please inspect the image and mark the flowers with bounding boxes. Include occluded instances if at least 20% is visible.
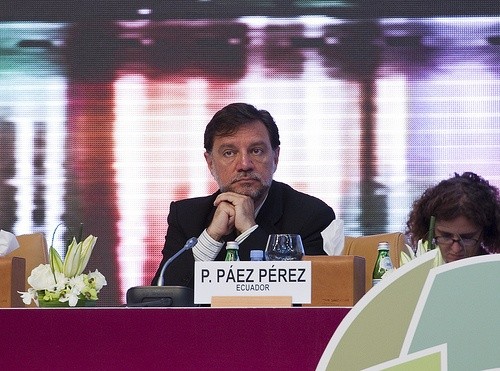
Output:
[24,222,107,307]
[400,239,428,268]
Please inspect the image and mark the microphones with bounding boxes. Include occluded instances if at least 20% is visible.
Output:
[127,236,196,307]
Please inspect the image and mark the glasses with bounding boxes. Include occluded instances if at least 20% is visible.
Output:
[430,229,483,247]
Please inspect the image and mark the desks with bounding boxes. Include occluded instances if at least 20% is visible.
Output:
[0,306,356,371]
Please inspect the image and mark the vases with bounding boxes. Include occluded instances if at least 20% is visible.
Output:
[35,290,88,306]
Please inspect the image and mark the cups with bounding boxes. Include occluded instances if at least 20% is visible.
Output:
[265,234,304,261]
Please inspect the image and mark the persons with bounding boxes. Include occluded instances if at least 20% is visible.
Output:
[151,103,336,287]
[406,171,500,263]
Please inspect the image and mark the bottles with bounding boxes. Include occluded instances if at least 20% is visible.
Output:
[250,250,263,261]
[373,243,393,286]
[224,241,240,262]
